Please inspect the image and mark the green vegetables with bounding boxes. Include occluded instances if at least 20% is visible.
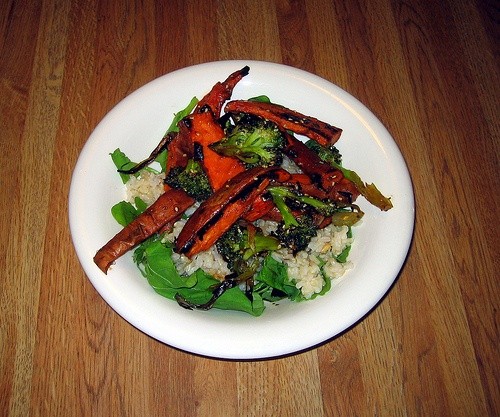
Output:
[108,97,394,315]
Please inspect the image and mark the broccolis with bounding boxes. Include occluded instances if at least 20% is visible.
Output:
[267,185,336,256]
[216,222,281,270]
[162,156,212,200]
[208,114,282,168]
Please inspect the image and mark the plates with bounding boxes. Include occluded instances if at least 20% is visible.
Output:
[68,60,416,360]
